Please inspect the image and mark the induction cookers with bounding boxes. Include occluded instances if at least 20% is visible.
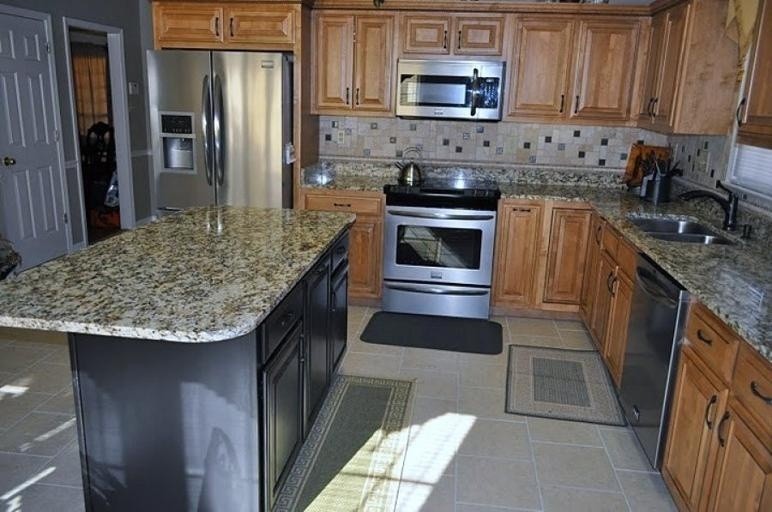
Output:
[383,180,500,210]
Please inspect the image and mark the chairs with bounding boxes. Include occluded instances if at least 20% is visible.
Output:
[84,122,119,173]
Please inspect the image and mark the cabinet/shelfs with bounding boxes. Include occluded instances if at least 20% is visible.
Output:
[299,190,380,301]
[488,198,544,317]
[397,8,505,59]
[637,1,735,141]
[296,256,329,439]
[152,3,296,50]
[68,286,303,511]
[660,291,771,511]
[303,7,397,117]
[577,215,644,391]
[734,1,772,153]
[508,14,647,124]
[536,198,590,312]
[324,229,354,379]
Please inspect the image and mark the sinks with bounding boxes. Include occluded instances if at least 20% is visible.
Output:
[647,234,730,245]
[627,212,706,233]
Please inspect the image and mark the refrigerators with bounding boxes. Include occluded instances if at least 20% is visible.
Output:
[144,49,293,226]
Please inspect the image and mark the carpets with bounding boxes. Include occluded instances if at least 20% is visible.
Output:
[503,343,628,429]
[264,371,418,511]
[359,308,504,357]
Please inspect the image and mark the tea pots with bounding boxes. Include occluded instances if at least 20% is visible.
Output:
[394,147,427,183]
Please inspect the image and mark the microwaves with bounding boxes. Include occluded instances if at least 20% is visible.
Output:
[396,58,504,122]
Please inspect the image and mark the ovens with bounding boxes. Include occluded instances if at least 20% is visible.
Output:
[381,205,495,320]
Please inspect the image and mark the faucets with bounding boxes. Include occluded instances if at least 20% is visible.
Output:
[677,180,739,230]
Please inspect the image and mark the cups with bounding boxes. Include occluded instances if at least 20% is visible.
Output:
[644,180,672,204]
